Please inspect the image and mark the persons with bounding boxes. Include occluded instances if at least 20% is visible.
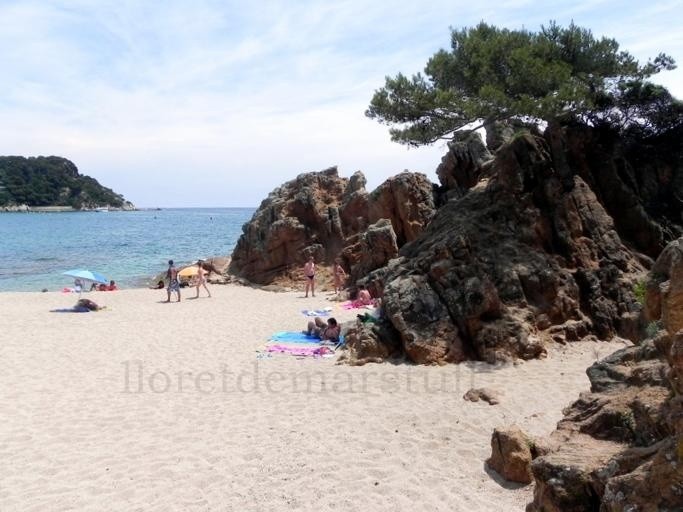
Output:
[303,255,316,298]
[163,260,181,304]
[299,315,340,343]
[193,261,210,300]
[62,278,117,295]
[185,275,199,287]
[354,284,371,306]
[330,258,347,294]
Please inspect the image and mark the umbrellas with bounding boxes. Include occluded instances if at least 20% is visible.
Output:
[60,269,109,286]
[175,265,209,277]
